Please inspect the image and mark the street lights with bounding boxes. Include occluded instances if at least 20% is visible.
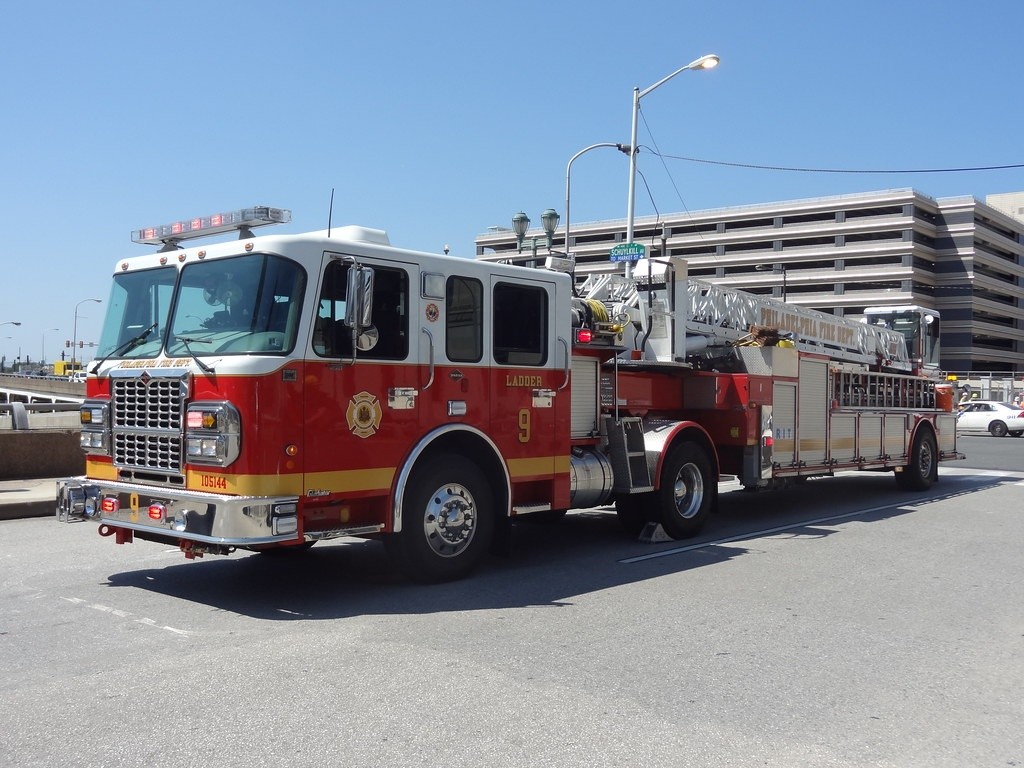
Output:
[624,54,721,279]
[41,328,60,367]
[71,298,103,382]
[512,209,561,269]
[565,143,640,254]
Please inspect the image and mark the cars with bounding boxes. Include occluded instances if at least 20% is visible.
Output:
[17,367,55,381]
[957,400,1024,437]
[68,372,87,383]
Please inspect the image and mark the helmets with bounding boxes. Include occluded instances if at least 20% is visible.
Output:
[1014,397,1020,401]
[972,393,977,397]
[962,392,968,396]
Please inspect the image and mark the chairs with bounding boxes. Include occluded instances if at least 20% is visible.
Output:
[976,405,986,411]
[342,310,406,357]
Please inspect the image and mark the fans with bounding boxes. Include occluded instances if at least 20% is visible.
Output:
[217,279,244,306]
[202,277,227,306]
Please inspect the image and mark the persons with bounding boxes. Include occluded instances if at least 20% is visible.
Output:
[959,392,977,411]
[1015,397,1021,407]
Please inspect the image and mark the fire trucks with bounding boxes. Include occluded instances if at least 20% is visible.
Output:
[55,205,975,586]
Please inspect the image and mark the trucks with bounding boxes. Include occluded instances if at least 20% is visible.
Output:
[53,360,85,377]
